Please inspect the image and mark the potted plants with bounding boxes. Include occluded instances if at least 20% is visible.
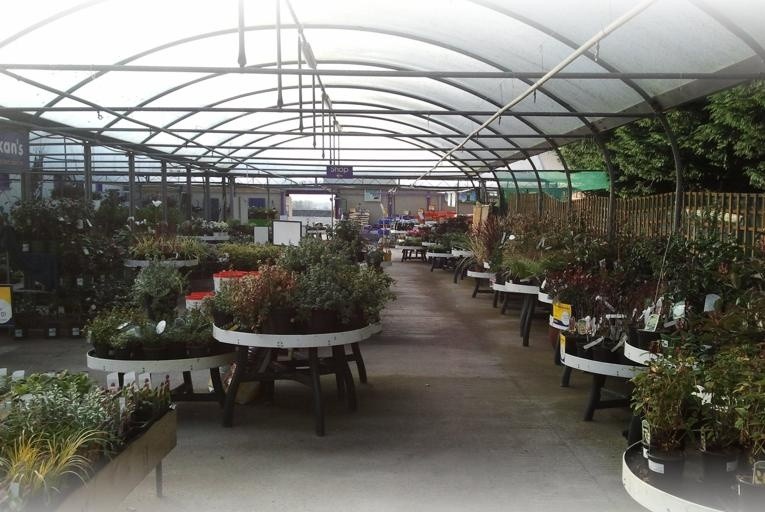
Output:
[0,218,398,362]
[627,322,765,495]
[402,214,501,281]
[1,373,182,511]
[502,218,764,370]
